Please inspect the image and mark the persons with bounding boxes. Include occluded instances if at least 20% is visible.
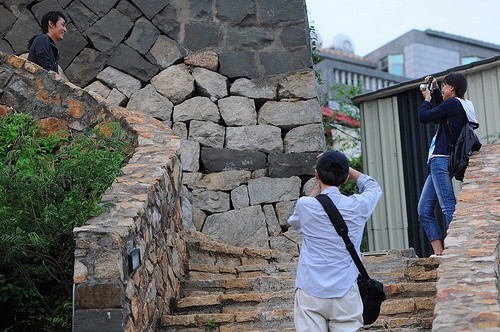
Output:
[417,73,479,258]
[287,151,382,332]
[27,10,68,75]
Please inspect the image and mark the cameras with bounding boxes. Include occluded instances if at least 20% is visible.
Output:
[420,77,433,92]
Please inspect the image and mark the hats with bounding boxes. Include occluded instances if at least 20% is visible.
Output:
[318,150,349,173]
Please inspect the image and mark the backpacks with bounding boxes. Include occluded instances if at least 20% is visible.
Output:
[449,122,482,182]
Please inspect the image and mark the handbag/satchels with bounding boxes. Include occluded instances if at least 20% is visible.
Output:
[357,274,387,325]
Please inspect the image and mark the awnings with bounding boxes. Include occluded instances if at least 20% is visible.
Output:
[320,105,360,141]
